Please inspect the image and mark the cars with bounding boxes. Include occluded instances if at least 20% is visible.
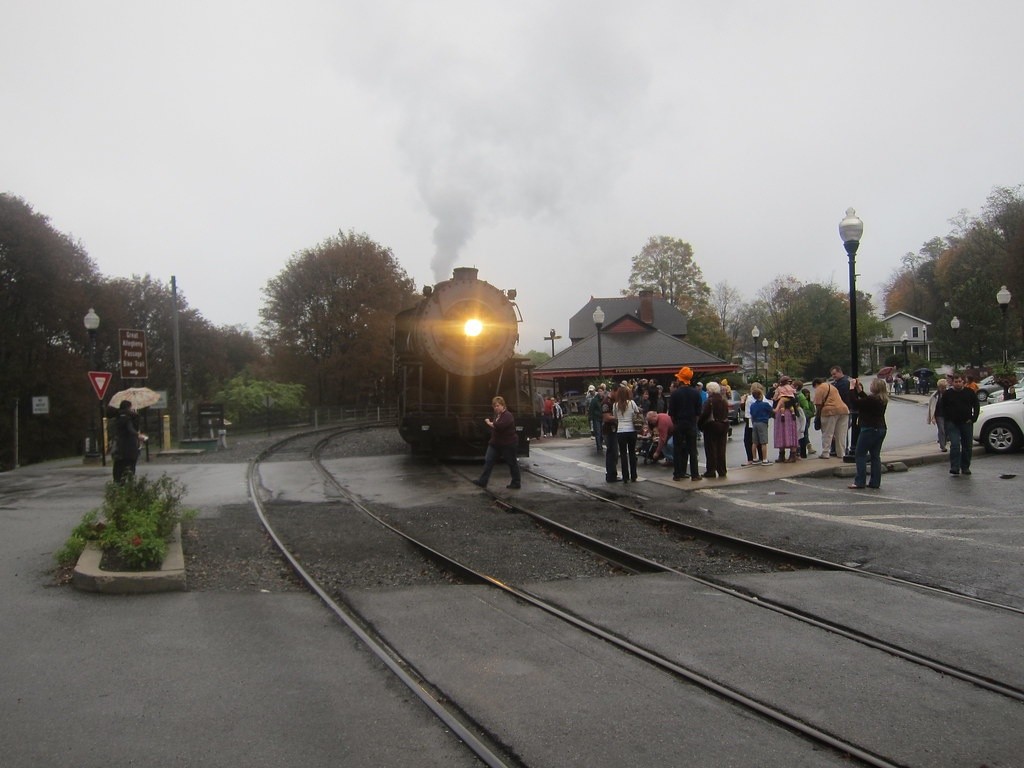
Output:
[727,391,745,424]
[976,362,1024,403]
[973,395,1024,453]
[864,369,873,375]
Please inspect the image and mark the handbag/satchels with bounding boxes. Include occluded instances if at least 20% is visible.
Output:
[814,414,821,430]
[703,398,715,426]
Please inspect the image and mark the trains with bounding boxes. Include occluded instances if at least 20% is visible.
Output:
[392,267,536,461]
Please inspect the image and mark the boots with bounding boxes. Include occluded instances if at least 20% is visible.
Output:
[785,452,796,463]
[775,452,785,463]
[807,444,816,454]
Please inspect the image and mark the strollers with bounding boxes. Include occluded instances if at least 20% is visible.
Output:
[635,436,665,465]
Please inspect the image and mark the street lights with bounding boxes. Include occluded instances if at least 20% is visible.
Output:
[550,329,556,398]
[996,285,1011,401]
[900,332,910,394]
[839,207,864,462]
[922,325,926,359]
[774,342,780,382]
[84,308,101,457]
[762,339,768,395]
[593,306,607,383]
[751,326,759,378]
[951,317,959,372]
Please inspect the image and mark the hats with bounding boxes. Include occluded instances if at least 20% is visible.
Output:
[721,379,727,385]
[697,382,703,387]
[674,367,693,385]
[588,385,595,391]
[781,376,792,382]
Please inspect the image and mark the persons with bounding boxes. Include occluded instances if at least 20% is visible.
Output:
[530,365,1016,489]
[470,396,520,489]
[107,400,146,483]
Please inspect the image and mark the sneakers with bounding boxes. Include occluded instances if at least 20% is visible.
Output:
[752,461,762,465]
[762,461,773,465]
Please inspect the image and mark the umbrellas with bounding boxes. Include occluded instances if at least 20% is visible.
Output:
[877,366,892,377]
[912,368,934,377]
[109,387,160,413]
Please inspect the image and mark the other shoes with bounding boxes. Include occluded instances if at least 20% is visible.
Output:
[591,436,595,440]
[848,483,858,488]
[607,477,622,482]
[507,484,520,488]
[673,473,726,481]
[663,461,672,467]
[472,480,486,487]
[741,461,752,466]
[942,448,947,451]
[950,470,959,476]
[867,485,879,488]
[819,455,829,459]
[962,470,971,474]
